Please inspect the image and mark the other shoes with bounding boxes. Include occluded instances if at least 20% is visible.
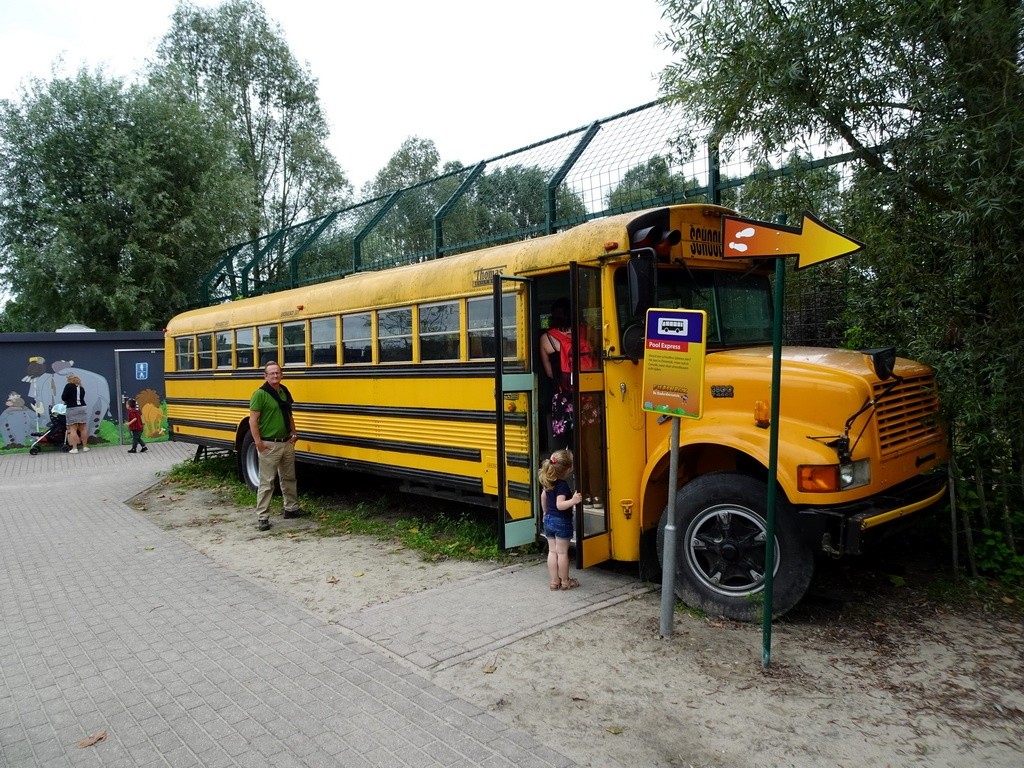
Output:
[83,446,89,451]
[583,497,592,509]
[69,448,78,453]
[593,496,602,509]
[140,447,147,452]
[128,448,136,453]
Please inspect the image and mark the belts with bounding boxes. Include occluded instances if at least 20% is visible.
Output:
[261,436,289,442]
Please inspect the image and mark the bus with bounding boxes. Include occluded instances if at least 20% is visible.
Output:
[162,204,951,624]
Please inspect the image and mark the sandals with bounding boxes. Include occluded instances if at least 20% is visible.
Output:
[550,577,561,591]
[560,577,580,590]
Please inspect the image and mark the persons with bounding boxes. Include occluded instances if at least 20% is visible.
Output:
[61,375,90,454]
[539,302,604,510]
[248,360,312,531]
[123,399,148,453]
[538,449,583,592]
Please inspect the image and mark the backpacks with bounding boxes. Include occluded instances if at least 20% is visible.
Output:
[547,323,599,394]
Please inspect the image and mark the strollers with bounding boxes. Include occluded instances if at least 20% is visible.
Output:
[29,403,72,455]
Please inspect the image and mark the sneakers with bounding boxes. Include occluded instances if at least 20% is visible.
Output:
[284,509,312,519]
[259,520,270,531]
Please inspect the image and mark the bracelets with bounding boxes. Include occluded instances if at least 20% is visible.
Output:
[292,434,298,441]
